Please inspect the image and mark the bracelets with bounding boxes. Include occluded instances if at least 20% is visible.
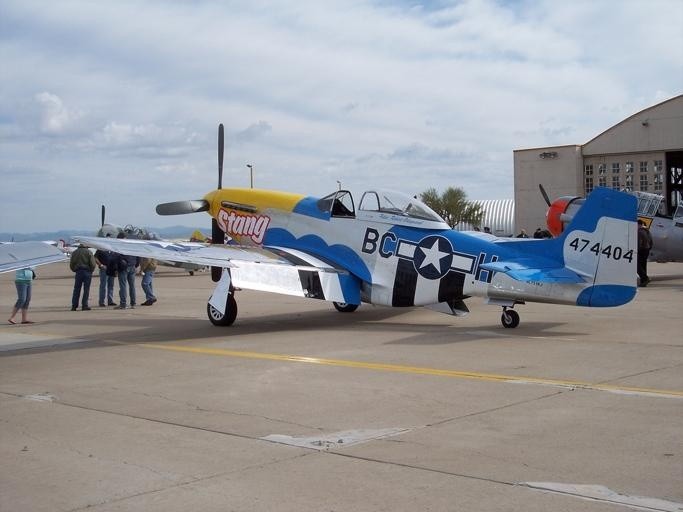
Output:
[99,264,103,267]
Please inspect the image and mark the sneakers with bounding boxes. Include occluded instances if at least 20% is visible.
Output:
[141,298,156,306]
[70,302,136,311]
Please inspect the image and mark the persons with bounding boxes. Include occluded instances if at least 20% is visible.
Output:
[67,243,96,312]
[114,231,140,311]
[92,232,117,307]
[516,228,529,238]
[533,228,544,238]
[6,265,35,326]
[473,226,480,232]
[137,233,157,307]
[636,219,654,288]
[483,226,492,234]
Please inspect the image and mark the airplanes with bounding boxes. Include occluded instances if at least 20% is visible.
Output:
[538,182,683,263]
